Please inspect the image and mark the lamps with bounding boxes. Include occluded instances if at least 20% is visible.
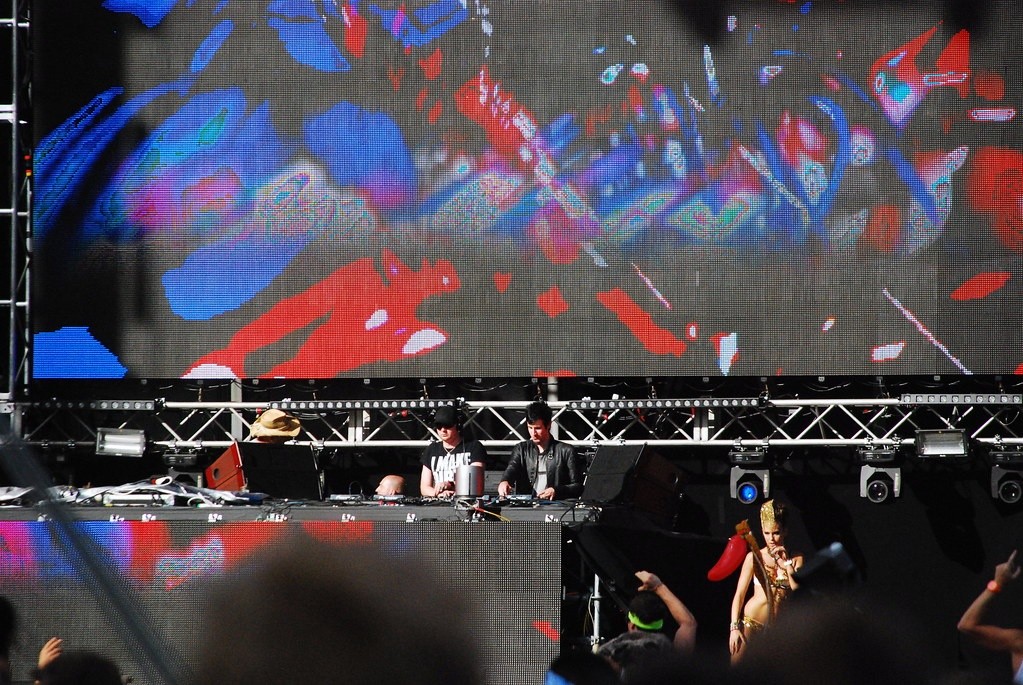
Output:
[728,437,1023,507]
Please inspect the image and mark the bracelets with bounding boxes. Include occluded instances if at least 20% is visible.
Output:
[784,560,792,567]
[730,622,739,630]
[448,481,455,491]
[987,580,1001,593]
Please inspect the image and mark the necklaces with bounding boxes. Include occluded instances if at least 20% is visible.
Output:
[443,439,461,458]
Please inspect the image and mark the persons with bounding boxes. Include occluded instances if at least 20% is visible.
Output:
[496,402,582,502]
[374,475,407,496]
[728,498,806,668]
[957,549,1023,685]
[420,407,488,500]
[2,533,963,685]
[246,408,301,444]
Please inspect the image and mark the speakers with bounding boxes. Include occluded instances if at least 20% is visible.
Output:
[204,438,322,501]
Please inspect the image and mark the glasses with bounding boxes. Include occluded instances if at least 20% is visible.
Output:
[435,423,454,430]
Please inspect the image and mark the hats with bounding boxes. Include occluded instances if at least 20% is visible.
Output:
[250,409,301,437]
[434,406,459,425]
